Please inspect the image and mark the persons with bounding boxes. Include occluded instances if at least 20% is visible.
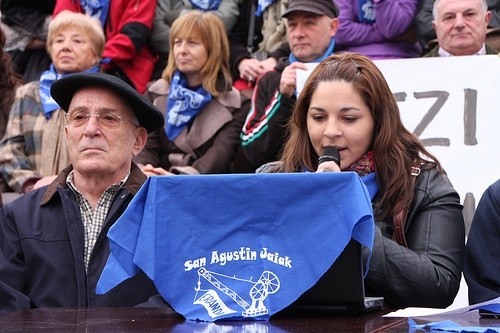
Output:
[52,0,159,96]
[462,178,500,310]
[0,10,106,195]
[132,9,251,177]
[415,0,500,59]
[149,0,239,85]
[227,0,291,98]
[0,28,25,192]
[239,0,340,165]
[0,71,165,305]
[254,52,464,308]
[0,0,57,86]
[332,0,422,60]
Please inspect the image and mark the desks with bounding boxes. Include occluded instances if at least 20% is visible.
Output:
[0,306,500,333]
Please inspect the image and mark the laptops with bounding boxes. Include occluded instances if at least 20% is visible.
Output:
[272,240,383,316]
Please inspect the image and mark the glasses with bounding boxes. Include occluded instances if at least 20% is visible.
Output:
[64,110,141,130]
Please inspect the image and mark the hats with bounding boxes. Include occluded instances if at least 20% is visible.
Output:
[50,73,164,133]
[281,0,340,19]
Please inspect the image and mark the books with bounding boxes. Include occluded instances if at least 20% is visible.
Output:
[478,309,500,317]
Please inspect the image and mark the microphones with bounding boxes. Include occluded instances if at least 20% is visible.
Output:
[318,148,341,167]
